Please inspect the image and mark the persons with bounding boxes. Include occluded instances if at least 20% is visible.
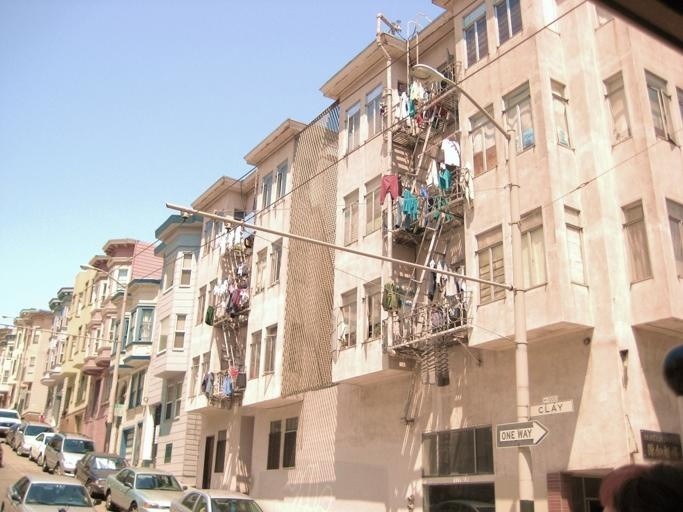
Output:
[598,346,682,512]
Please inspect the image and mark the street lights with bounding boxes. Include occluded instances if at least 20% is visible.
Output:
[1,314,31,411]
[407,57,536,512]
[78,262,137,457]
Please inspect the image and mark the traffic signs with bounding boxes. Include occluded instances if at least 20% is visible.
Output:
[494,419,549,448]
[529,399,576,418]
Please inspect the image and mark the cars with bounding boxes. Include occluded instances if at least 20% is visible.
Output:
[0,407,188,512]
[169,487,264,512]
[428,499,494,512]
